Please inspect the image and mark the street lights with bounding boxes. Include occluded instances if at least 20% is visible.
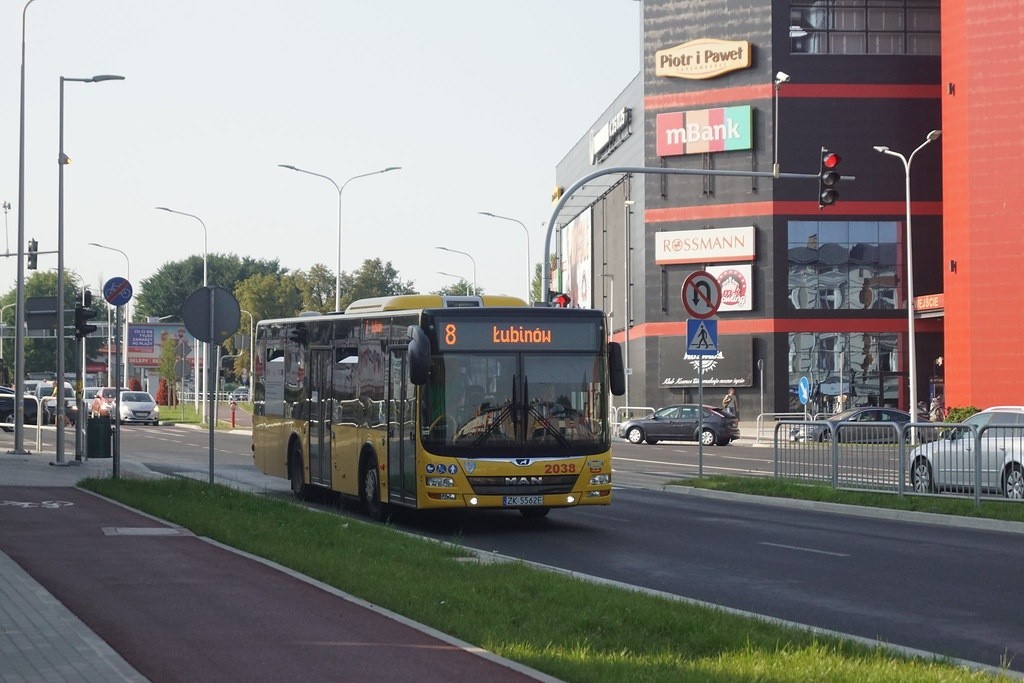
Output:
[276,163,403,312]
[48,268,87,388]
[88,242,130,388]
[438,271,471,296]
[874,128,943,445]
[47,74,128,468]
[155,206,210,424]
[435,245,477,296]
[478,212,532,307]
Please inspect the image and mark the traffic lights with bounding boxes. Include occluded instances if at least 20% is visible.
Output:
[817,145,841,207]
[549,291,572,308]
[74,304,98,340]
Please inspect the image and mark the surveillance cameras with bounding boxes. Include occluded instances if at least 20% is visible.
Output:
[776,71,791,82]
[625,201,635,205]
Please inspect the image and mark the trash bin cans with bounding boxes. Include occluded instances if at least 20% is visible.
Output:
[86,416,112,458]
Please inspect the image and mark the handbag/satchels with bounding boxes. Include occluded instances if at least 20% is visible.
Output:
[722,395,731,407]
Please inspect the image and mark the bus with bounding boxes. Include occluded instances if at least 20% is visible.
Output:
[251,296,625,523]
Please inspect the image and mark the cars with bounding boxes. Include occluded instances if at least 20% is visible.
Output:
[0,370,132,433]
[795,406,938,446]
[229,385,248,401]
[618,403,741,446]
[109,391,160,426]
[909,405,1024,500]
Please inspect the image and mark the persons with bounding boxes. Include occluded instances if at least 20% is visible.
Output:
[50,381,72,428]
[930,394,947,434]
[419,356,470,437]
[722,387,738,417]
[527,378,566,441]
[11,383,16,390]
